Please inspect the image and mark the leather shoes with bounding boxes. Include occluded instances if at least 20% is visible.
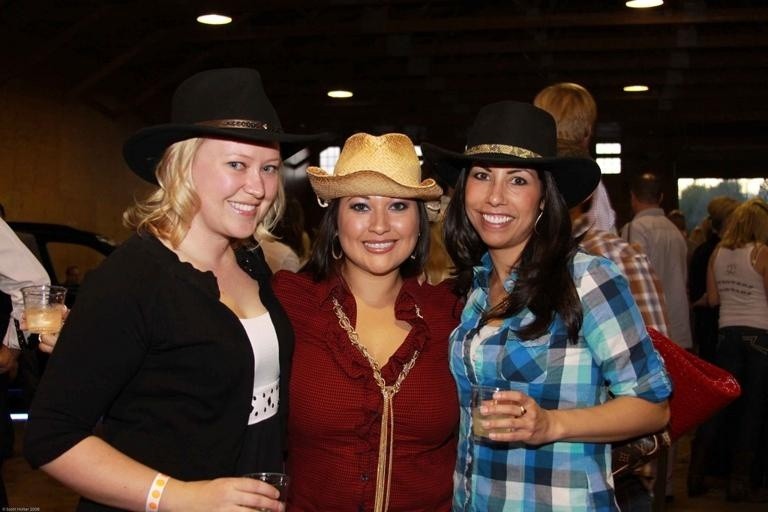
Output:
[689,486,707,498]
[709,478,725,484]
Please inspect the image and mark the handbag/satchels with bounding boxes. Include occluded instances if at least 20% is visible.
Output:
[564,244,671,512]
[646,325,741,441]
[1,291,12,353]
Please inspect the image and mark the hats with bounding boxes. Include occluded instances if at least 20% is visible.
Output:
[306,132,445,200]
[421,100,602,211]
[122,69,338,188]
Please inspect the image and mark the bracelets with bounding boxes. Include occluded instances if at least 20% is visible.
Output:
[142,471,171,512]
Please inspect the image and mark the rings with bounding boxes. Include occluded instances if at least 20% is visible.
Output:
[515,405,528,418]
[39,332,49,342]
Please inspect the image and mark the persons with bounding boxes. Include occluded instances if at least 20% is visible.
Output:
[619,172,694,512]
[0,212,52,512]
[668,208,687,229]
[687,196,741,489]
[447,102,672,512]
[22,66,296,512]
[708,201,768,505]
[270,132,466,512]
[687,240,717,496]
[533,83,596,158]
[547,155,669,512]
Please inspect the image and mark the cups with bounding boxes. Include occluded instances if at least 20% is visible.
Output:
[244,473,289,512]
[20,285,66,336]
[472,385,510,442]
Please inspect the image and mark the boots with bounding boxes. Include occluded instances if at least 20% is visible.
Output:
[727,475,753,502]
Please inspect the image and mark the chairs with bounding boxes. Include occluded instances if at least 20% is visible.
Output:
[6,221,120,286]
